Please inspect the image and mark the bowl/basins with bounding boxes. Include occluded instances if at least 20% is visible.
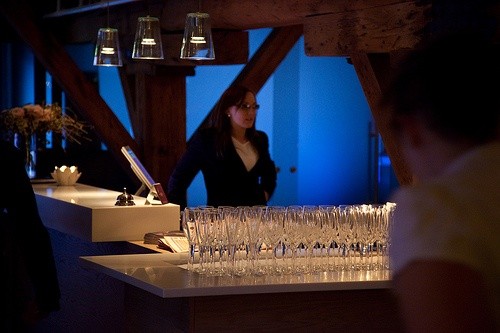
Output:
[51,172,80,186]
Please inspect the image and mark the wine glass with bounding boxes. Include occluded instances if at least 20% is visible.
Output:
[180,203,396,278]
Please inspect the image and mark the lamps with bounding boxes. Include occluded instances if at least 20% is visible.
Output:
[132,0,165,60]
[179,0,216,60]
[93,0,124,68]
[0,101,95,145]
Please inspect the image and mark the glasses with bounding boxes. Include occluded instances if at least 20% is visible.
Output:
[234,103,259,110]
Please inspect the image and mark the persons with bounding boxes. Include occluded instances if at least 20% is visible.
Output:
[0,140,62,333]
[380,45,500,333]
[165,85,277,207]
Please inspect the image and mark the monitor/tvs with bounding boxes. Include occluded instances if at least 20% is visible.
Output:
[121,146,164,205]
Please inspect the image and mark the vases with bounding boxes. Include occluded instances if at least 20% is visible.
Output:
[20,126,37,180]
